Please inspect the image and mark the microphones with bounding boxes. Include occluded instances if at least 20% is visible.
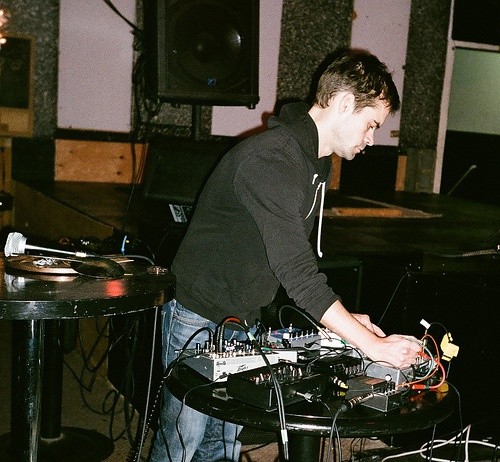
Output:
[4,232,96,259]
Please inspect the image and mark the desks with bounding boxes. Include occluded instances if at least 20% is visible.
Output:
[0,252,176,462]
[165,341,459,462]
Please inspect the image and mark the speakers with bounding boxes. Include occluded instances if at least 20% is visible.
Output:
[143,0,260,110]
[143,136,236,205]
